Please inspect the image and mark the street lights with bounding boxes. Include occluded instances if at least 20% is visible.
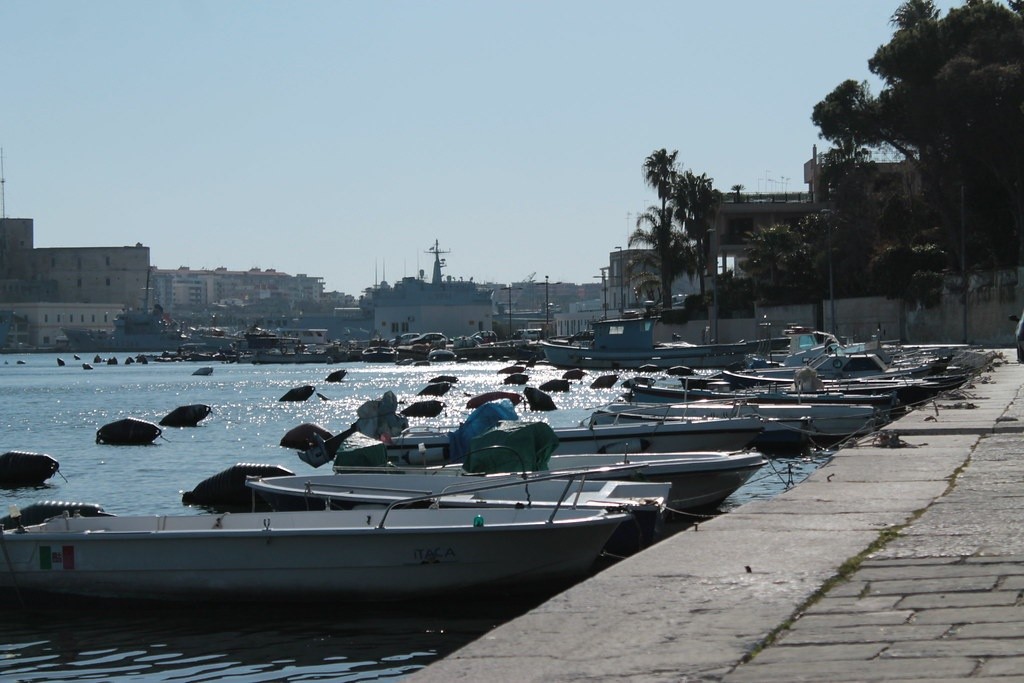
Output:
[500,285,523,339]
[536,276,561,338]
[615,247,624,318]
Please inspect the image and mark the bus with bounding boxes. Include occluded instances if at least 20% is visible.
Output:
[515,328,543,341]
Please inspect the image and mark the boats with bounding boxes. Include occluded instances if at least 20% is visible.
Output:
[51,300,1009,453]
[0,450,636,620]
[332,444,773,530]
[244,465,674,579]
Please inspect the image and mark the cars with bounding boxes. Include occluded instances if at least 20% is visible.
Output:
[389,332,420,346]
[468,330,498,344]
[409,332,448,346]
[1008,308,1024,365]
